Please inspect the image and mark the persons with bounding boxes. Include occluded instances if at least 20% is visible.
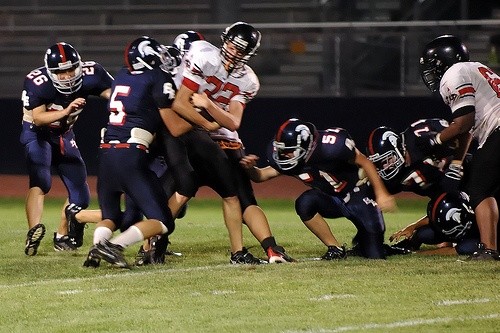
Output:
[364,35,500,261]
[15,42,114,257]
[64,22,295,268]
[238,117,411,259]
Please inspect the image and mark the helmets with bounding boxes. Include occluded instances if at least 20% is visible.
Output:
[427,190,476,241]
[366,125,407,181]
[163,30,206,77]
[272,118,320,175]
[418,34,470,95]
[124,36,173,75]
[44,42,84,96]
[219,22,262,74]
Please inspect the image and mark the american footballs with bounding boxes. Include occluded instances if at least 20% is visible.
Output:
[190,99,207,117]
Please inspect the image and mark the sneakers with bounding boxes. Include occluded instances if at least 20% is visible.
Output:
[83,245,101,268]
[64,203,87,247]
[267,245,297,263]
[229,246,269,264]
[54,231,81,257]
[24,224,46,256]
[95,238,131,271]
[314,242,348,261]
[135,235,175,268]
[457,241,500,262]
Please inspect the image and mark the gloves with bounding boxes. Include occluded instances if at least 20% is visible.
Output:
[419,131,446,160]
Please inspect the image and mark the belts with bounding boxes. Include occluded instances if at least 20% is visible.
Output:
[99,143,149,153]
